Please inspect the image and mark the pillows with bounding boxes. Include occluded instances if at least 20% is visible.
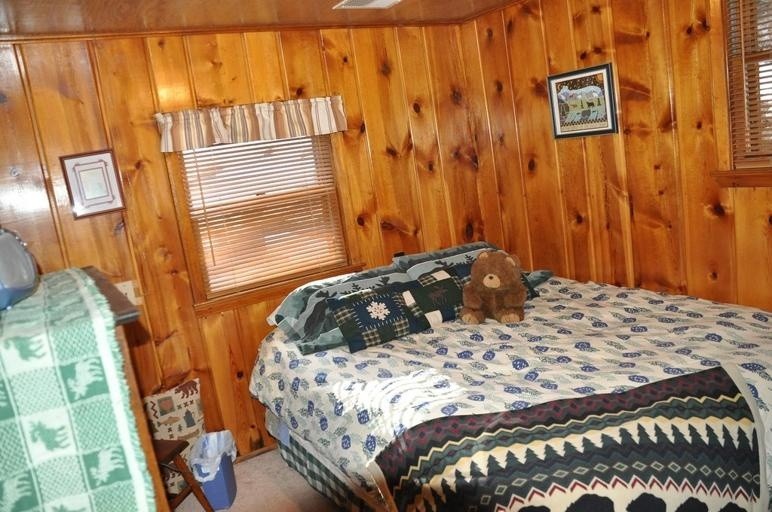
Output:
[139,377,206,495]
[266,241,553,352]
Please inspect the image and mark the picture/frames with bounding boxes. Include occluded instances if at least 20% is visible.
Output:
[545,62,619,138]
[57,149,127,220]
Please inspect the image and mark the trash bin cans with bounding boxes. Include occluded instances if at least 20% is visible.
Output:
[188,430,238,509]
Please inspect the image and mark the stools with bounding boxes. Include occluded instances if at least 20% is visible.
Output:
[153,440,214,512]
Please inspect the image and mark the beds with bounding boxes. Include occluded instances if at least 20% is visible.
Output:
[276,271,772,511]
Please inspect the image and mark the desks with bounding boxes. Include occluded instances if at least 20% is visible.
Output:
[1,267,170,512]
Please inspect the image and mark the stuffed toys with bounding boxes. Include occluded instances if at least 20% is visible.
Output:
[460,249,526,327]
